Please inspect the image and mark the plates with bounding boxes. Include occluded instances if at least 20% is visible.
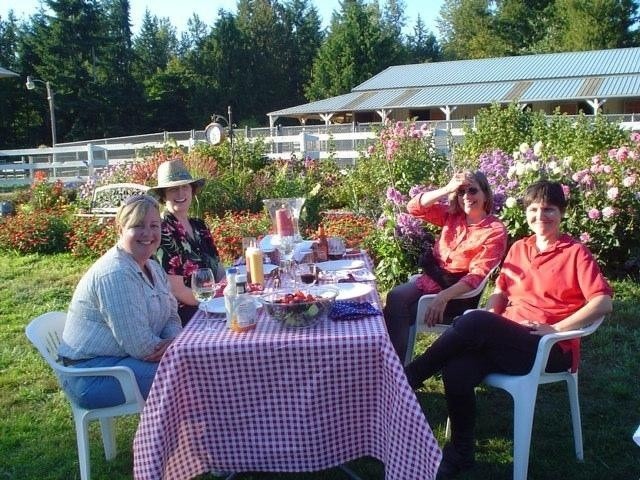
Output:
[198,295,264,315]
[299,285,373,307]
[316,260,368,273]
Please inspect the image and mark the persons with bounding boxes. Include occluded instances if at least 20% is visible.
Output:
[404,181,615,478]
[383,169,507,367]
[54,196,182,407]
[146,160,225,328]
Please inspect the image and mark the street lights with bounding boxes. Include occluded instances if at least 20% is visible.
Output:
[25,75,58,178]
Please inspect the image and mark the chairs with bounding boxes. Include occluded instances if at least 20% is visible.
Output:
[401,258,503,381]
[25,310,145,480]
[443,294,609,479]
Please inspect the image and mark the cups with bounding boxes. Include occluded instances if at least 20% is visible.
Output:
[299,250,314,263]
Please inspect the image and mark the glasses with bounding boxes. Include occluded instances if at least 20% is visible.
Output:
[456,186,483,198]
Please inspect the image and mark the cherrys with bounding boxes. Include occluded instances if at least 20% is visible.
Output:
[274,291,322,314]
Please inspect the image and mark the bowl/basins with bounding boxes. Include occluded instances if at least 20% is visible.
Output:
[261,288,338,328]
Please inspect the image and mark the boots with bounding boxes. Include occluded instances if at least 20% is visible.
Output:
[436,404,479,479]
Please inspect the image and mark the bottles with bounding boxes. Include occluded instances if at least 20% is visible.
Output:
[316,221,327,262]
[223,267,259,333]
[244,240,266,288]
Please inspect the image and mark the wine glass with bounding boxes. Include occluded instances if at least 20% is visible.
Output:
[328,238,345,284]
[190,269,217,336]
[278,259,293,297]
[299,263,317,303]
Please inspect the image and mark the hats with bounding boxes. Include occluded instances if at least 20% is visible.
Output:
[146,158,208,203]
